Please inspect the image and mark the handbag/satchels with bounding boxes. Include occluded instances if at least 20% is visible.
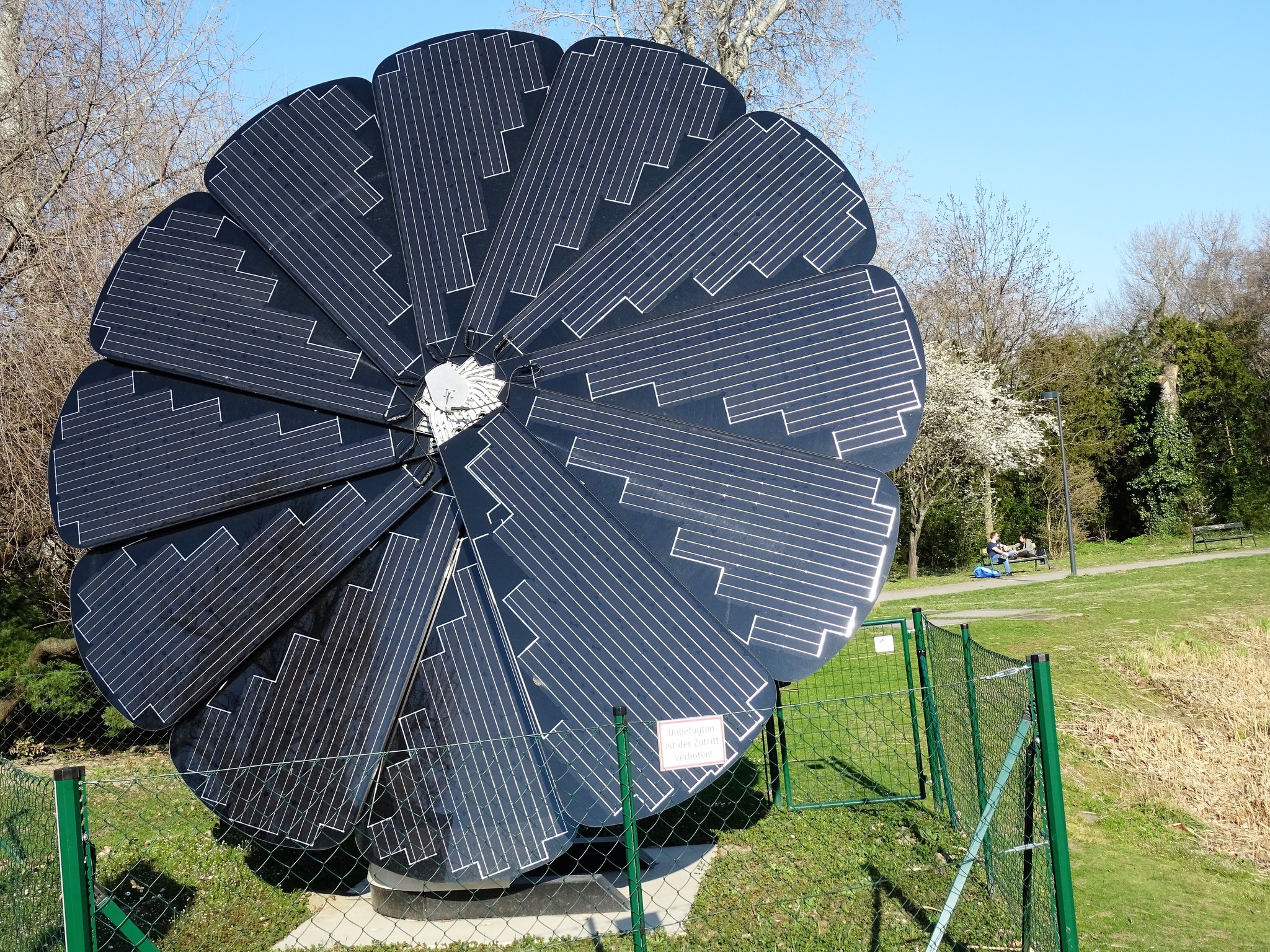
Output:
[1035,548,1044,556]
[974,565,1002,578]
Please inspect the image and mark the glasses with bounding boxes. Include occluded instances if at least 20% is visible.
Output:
[993,535,999,537]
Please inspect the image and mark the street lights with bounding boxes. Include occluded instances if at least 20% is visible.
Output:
[1039,391,1077,576]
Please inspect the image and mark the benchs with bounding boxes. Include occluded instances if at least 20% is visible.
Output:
[981,543,1050,571]
[1191,522,1257,553]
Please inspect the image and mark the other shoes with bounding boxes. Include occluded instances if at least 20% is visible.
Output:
[1000,555,1007,560]
[1006,573,1012,576]
[1008,556,1017,560]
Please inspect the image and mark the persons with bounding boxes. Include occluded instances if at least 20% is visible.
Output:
[1009,534,1036,560]
[987,532,1014,576]
